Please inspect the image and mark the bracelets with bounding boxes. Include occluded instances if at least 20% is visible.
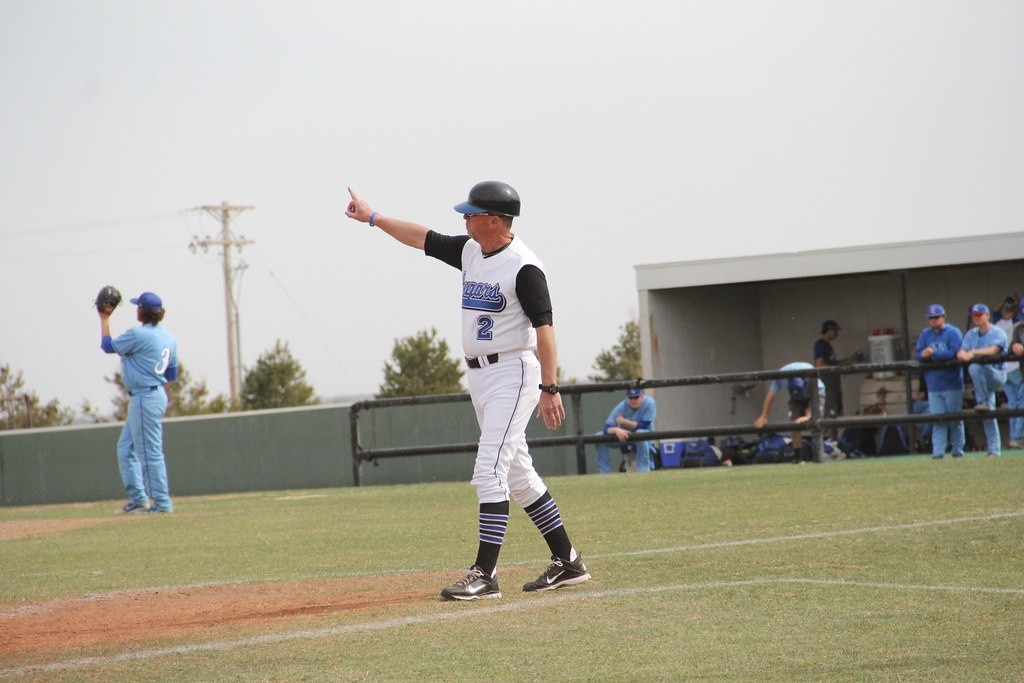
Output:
[369,211,377,226]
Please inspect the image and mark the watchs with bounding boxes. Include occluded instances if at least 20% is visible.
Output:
[538,384,560,395]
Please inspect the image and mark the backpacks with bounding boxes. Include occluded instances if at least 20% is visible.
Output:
[877,423,910,455]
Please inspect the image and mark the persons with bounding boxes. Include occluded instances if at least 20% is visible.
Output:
[957,304,1007,458]
[755,361,825,465]
[994,295,1024,449]
[346,182,588,602]
[101,293,180,514]
[914,305,966,461]
[812,321,863,443]
[594,389,657,474]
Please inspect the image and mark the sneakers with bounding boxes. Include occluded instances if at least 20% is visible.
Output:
[523,551,592,595]
[440,565,503,600]
[114,503,173,514]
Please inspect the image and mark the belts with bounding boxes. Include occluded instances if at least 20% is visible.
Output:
[465,353,499,368]
[128,385,158,396]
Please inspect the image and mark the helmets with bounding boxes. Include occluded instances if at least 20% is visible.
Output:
[453,180,520,218]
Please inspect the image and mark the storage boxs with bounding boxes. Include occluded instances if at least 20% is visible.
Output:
[867,333,903,379]
[659,438,710,467]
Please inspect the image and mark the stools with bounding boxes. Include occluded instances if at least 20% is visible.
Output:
[619,444,656,472]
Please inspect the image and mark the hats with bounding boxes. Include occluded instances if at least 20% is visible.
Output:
[970,304,990,314]
[130,292,163,311]
[924,304,946,318]
[789,378,805,401]
[627,388,644,397]
[822,320,843,331]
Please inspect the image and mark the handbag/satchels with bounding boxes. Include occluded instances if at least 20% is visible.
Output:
[682,433,864,469]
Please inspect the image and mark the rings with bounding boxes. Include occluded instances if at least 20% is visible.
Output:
[555,412,560,416]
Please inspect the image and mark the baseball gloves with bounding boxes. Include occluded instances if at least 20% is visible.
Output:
[96,285,123,316]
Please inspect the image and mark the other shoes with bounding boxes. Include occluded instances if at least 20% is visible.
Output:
[1008,439,1024,449]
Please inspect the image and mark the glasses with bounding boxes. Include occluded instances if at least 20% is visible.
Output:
[929,316,941,319]
[464,212,489,217]
[973,313,984,317]
[629,396,640,400]
